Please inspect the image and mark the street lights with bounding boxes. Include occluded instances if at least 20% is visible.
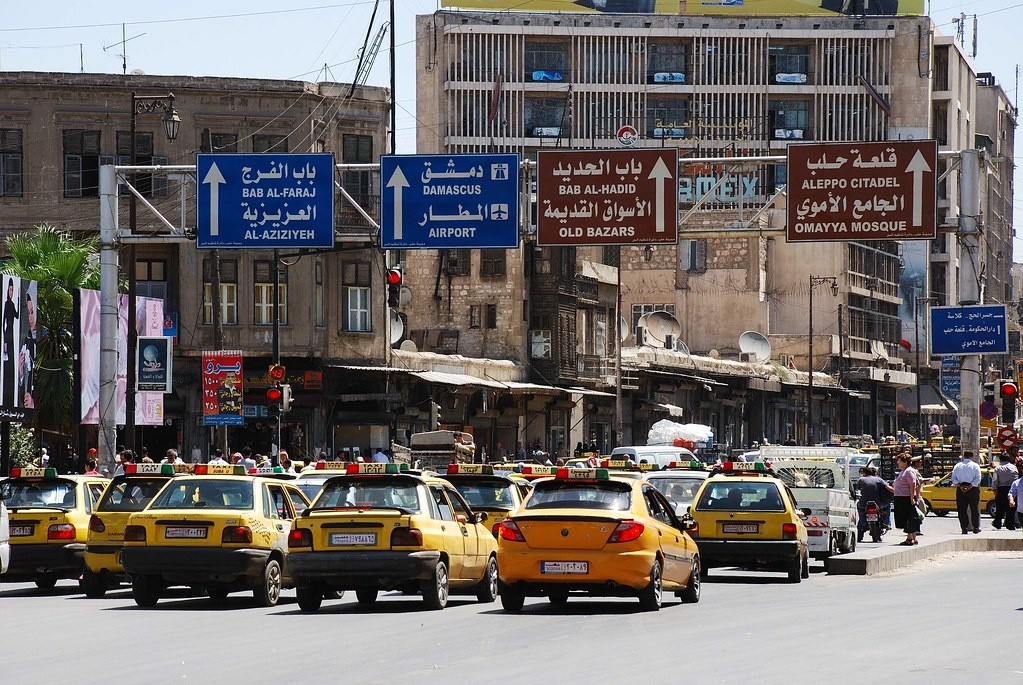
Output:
[806,275,839,446]
[126,91,182,451]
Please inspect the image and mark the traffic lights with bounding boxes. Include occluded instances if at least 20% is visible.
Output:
[268,364,285,383]
[266,386,281,428]
[1000,381,1019,425]
[984,379,1002,407]
[386,269,404,308]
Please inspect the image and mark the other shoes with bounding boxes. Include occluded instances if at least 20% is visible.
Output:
[962,528,967,534]
[882,521,891,530]
[973,527,981,533]
[916,531,922,535]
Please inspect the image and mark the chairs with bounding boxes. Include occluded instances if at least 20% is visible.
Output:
[713,498,734,507]
[63,491,73,503]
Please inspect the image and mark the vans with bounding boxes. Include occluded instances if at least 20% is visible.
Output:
[0,480,11,575]
[610,445,699,469]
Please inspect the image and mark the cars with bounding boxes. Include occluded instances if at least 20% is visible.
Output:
[84,450,811,612]
[919,460,997,517]
[837,431,1003,476]
[3,467,138,589]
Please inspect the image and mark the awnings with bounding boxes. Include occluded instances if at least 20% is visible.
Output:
[555,385,618,427]
[898,387,958,416]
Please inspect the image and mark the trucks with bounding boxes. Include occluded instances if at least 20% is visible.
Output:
[769,460,863,560]
[391,430,476,474]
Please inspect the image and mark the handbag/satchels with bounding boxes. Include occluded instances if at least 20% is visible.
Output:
[904,505,919,533]
[877,483,894,504]
[915,506,925,520]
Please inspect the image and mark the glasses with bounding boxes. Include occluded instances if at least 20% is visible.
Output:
[1013,450,1018,452]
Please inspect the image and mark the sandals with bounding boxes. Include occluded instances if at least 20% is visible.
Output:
[900,537,918,545]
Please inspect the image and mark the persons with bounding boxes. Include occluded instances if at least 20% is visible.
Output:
[951,450,982,535]
[891,453,933,546]
[496,441,507,460]
[335,450,345,460]
[453,431,477,449]
[555,441,570,456]
[38,444,101,475]
[19,279,38,409]
[763,434,797,446]
[2,278,20,406]
[990,446,1023,530]
[574,441,584,458]
[363,448,392,464]
[300,457,318,472]
[114,445,186,477]
[876,420,947,442]
[191,444,297,473]
[623,454,637,464]
[516,441,526,460]
[319,452,327,462]
[854,467,895,543]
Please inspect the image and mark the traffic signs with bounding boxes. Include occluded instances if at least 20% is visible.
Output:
[380,151,519,249]
[537,144,679,246]
[195,151,335,248]
[783,140,936,242]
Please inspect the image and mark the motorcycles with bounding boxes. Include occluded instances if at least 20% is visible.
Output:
[850,495,892,543]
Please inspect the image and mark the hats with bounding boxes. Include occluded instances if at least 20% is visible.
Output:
[89,448,96,454]
[911,455,922,461]
[280,451,288,459]
